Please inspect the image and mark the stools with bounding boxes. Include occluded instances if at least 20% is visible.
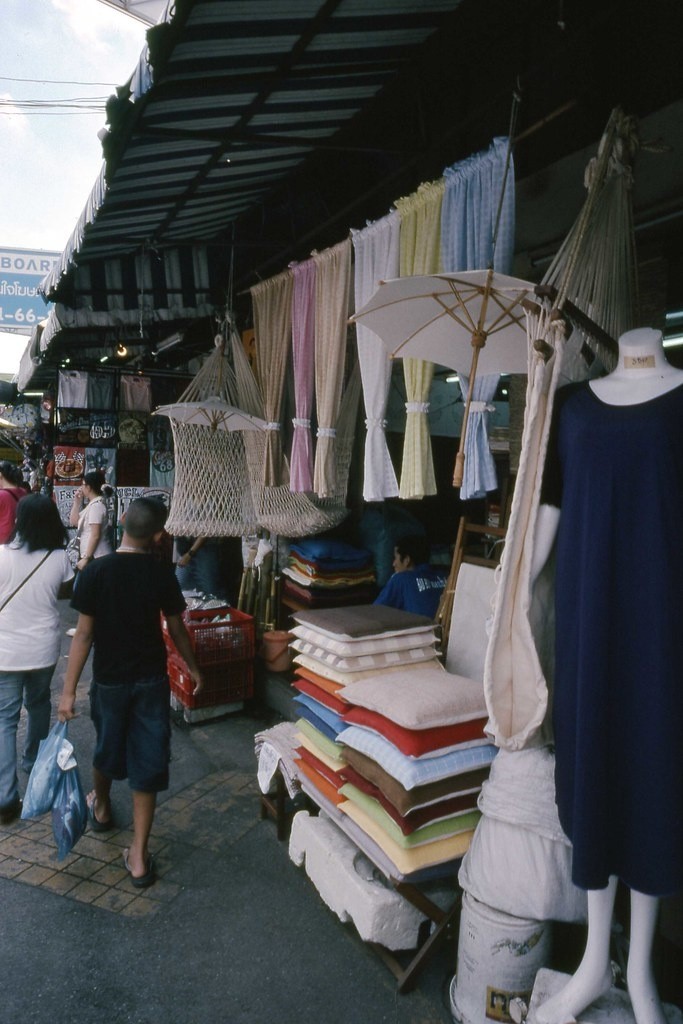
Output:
[258,769,312,842]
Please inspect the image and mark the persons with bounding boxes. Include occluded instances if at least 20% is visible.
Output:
[58,497,207,888]
[1,493,77,825]
[0,461,29,546]
[65,472,111,637]
[533,327,683,1024]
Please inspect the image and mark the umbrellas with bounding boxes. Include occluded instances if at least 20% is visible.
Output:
[346,269,539,490]
[147,400,275,432]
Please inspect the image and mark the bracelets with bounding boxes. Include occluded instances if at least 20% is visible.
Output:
[81,553,91,559]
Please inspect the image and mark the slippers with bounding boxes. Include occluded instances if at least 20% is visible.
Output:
[123,847,157,889]
[85,790,113,831]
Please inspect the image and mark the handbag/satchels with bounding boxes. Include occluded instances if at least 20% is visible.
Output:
[65,537,80,571]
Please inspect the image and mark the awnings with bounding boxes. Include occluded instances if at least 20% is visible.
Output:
[13,1,680,426]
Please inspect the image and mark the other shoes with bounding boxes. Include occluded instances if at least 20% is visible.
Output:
[0,798,24,826]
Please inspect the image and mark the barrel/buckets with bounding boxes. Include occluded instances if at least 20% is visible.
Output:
[263,630,296,673]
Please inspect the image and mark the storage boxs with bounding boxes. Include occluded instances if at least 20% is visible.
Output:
[161,605,257,708]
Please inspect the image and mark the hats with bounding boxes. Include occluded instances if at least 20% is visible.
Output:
[397,533,431,564]
